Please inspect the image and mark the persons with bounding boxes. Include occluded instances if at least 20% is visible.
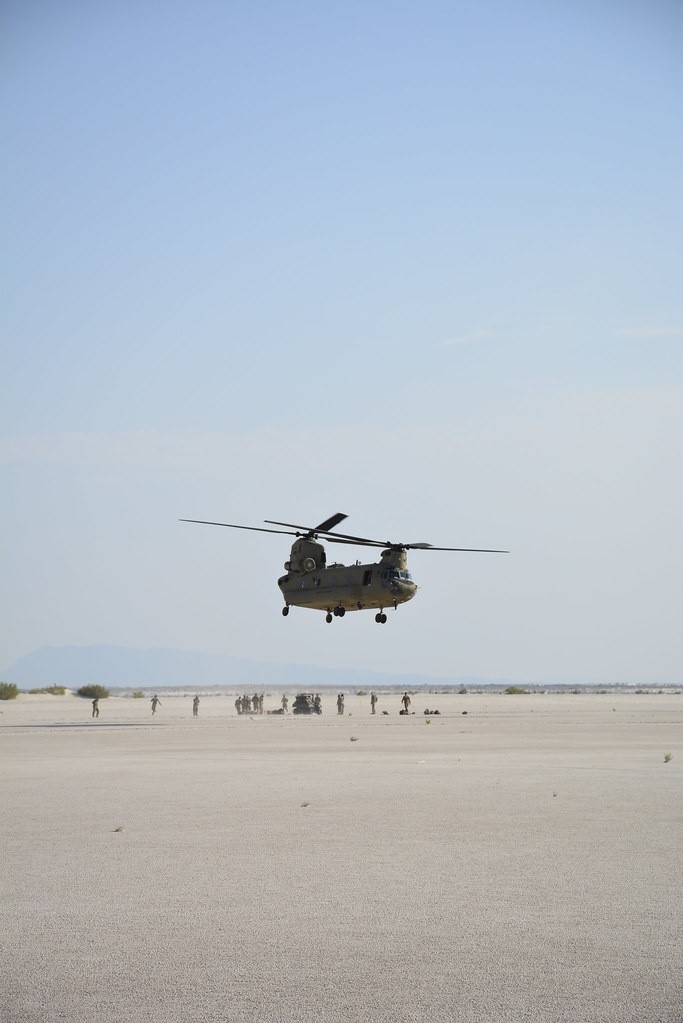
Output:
[402,692,411,711]
[193,696,200,716]
[371,692,375,714]
[315,694,320,702]
[235,693,264,714]
[281,695,288,712]
[92,697,99,717]
[151,695,161,715]
[337,694,344,715]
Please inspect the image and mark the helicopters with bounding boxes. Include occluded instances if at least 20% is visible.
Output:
[178,513,510,624]
